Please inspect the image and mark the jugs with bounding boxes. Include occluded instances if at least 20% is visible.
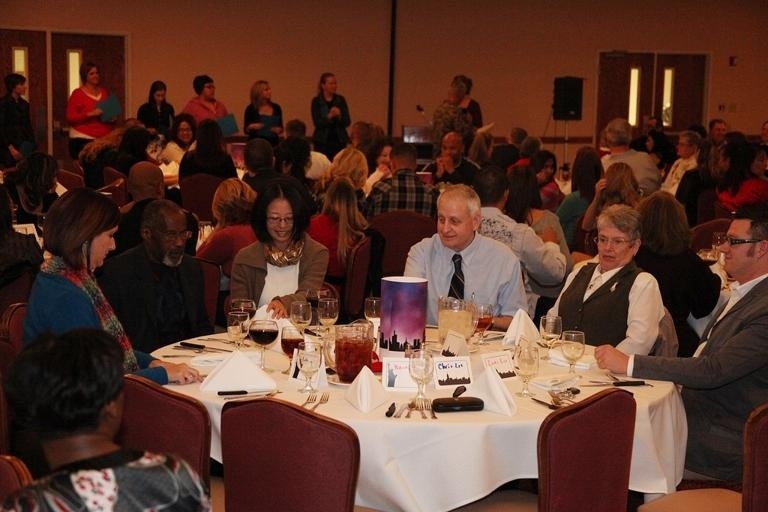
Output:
[326,324,375,381]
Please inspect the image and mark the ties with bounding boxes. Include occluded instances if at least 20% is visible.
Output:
[448,255,464,299]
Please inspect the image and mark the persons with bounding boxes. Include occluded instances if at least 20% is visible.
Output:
[594,201,768,511]
[65,63,117,159]
[457,76,483,130]
[138,81,174,128]
[311,73,351,163]
[0,74,35,168]
[244,81,283,148]
[0,114,767,383]
[0,328,210,512]
[433,75,475,157]
[182,76,227,126]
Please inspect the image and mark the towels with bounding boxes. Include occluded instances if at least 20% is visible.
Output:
[457,366,518,418]
[342,365,387,412]
[253,302,301,341]
[501,310,538,346]
[199,348,281,396]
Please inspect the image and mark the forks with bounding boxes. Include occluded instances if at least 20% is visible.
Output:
[300,392,330,410]
[417,399,438,421]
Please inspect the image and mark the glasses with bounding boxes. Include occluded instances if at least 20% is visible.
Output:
[724,236,758,246]
[593,237,634,247]
[155,230,192,241]
[266,216,294,225]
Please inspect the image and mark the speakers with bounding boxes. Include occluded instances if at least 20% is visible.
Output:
[552,75,583,121]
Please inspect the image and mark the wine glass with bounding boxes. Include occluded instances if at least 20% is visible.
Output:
[296,341,323,394]
[227,297,384,349]
[562,330,585,379]
[699,232,730,261]
[514,347,543,398]
[280,325,306,374]
[539,316,562,360]
[407,348,435,411]
[249,320,279,372]
[438,296,492,353]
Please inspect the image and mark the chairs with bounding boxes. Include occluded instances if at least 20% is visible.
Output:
[4,300,33,355]
[691,214,732,253]
[368,210,438,278]
[635,402,767,510]
[192,256,218,331]
[2,453,33,497]
[220,395,360,512]
[534,388,637,512]
[119,372,213,491]
[343,235,375,316]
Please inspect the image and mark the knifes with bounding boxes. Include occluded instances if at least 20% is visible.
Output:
[217,391,282,395]
[163,341,234,358]
[580,373,653,389]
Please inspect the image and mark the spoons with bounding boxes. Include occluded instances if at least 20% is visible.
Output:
[406,402,417,419]
